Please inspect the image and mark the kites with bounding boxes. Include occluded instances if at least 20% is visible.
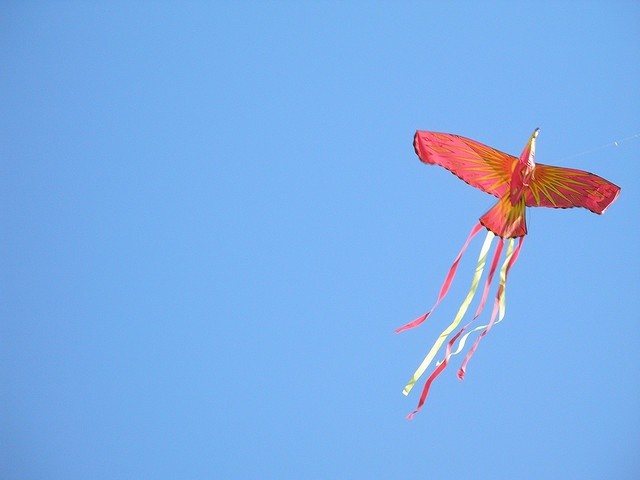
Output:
[395,127,622,420]
